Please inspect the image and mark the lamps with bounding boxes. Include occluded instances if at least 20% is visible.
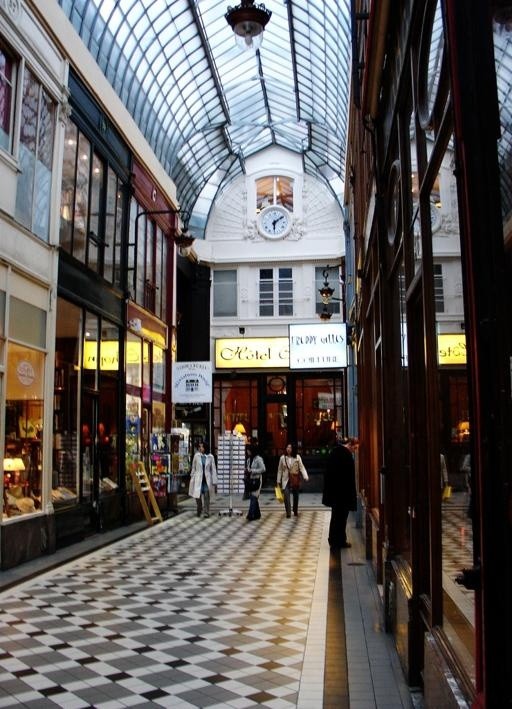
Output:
[319,295,342,322]
[125,208,197,288]
[315,262,343,304]
[220,1,271,51]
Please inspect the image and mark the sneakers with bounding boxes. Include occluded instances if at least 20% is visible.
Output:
[329,536,351,549]
[293,508,299,516]
[285,510,292,518]
[204,512,210,518]
[197,507,203,517]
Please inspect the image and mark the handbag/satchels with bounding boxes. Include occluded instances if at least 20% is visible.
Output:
[274,487,285,504]
[242,478,261,500]
[288,472,301,490]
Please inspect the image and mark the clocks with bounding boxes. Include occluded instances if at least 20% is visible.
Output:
[412,201,442,234]
[256,205,294,240]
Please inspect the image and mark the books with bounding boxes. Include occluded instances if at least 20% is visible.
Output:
[148,430,180,498]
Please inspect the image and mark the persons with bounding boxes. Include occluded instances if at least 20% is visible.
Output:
[321,436,360,550]
[460,451,472,498]
[242,442,267,521]
[187,441,220,518]
[440,453,451,521]
[452,419,470,444]
[276,440,310,519]
